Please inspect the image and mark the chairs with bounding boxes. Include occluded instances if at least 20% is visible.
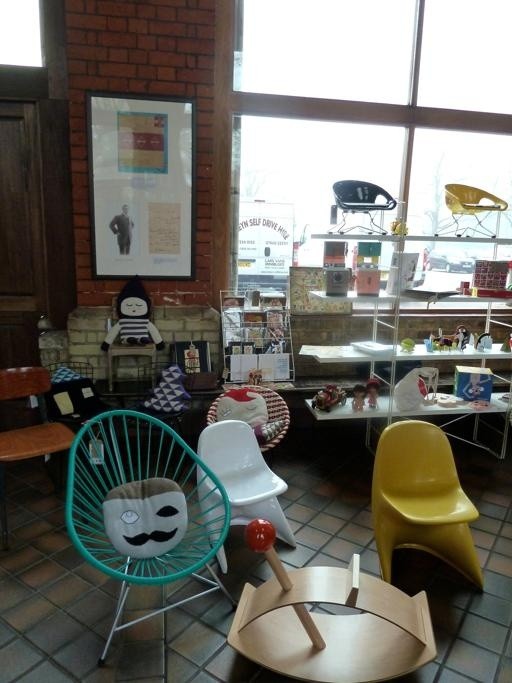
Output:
[32,362,119,497]
[0,366,77,552]
[65,409,238,666]
[197,419,297,574]
[120,362,195,481]
[435,183,508,238]
[207,385,291,452]
[372,420,483,595]
[333,180,397,236]
[106,296,157,390]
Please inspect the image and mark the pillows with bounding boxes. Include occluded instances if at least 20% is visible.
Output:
[216,389,282,442]
[40,367,111,434]
[104,477,188,559]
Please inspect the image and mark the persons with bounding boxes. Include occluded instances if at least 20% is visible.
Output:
[352,383,367,410]
[109,204,134,254]
[101,276,166,354]
[366,378,380,407]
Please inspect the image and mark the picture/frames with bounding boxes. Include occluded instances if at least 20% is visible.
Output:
[84,88,196,281]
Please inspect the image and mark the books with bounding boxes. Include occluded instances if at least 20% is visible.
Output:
[349,340,395,354]
[183,348,201,374]
[221,293,290,382]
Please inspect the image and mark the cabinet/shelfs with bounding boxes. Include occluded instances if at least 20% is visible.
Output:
[305,234,512,457]
